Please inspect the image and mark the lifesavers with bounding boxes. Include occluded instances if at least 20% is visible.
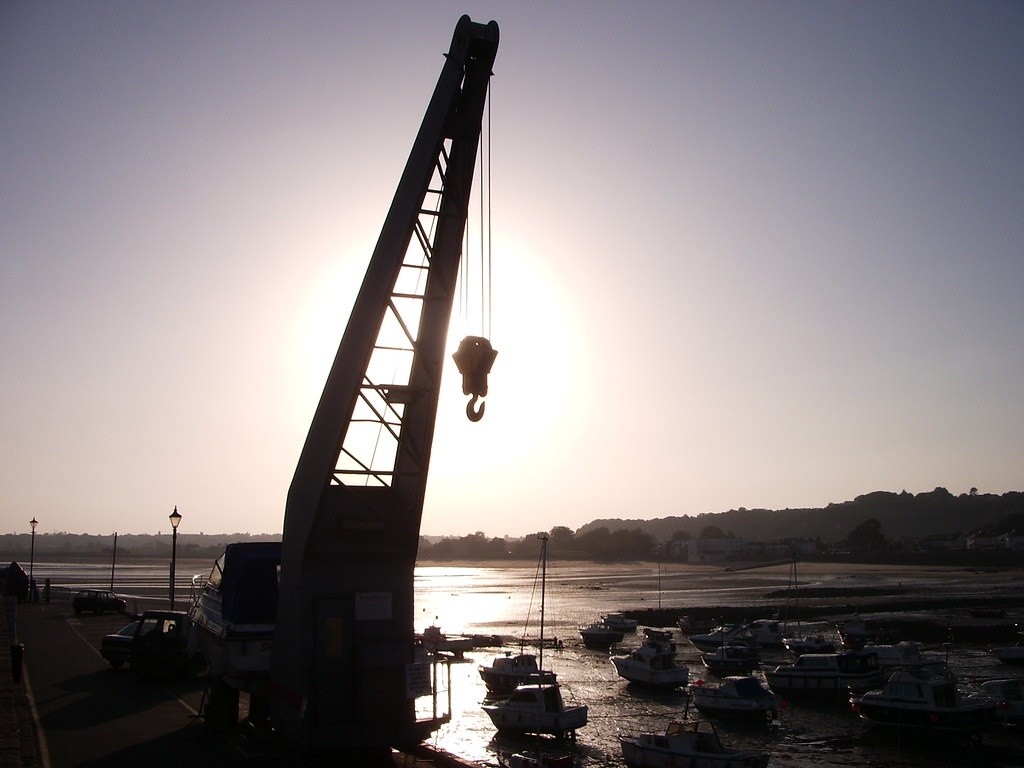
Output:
[819,635,823,640]
[905,657,911,662]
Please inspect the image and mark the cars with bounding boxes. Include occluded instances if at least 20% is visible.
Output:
[100,619,169,668]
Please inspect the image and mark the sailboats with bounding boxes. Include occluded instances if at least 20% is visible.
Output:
[480,532,587,735]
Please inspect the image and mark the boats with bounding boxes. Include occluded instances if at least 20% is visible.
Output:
[609,550,1024,730]
[601,612,638,631]
[578,623,624,645]
[617,686,772,768]
[479,653,557,695]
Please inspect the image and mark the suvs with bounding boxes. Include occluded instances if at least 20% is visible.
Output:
[73,589,127,615]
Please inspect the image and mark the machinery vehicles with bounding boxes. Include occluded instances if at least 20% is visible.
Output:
[129,12,502,768]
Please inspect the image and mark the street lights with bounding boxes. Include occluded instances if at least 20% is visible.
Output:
[170,505,183,611]
[28,518,37,603]
